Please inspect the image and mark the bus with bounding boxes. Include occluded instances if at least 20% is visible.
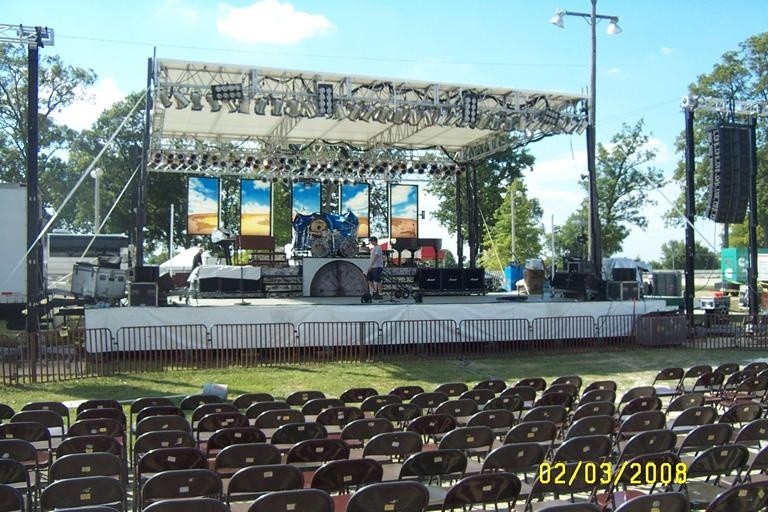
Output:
[49,229,127,274]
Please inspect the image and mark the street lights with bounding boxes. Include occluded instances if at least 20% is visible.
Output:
[553,0,624,289]
[86,167,109,236]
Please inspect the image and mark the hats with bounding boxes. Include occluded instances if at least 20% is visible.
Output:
[369,237,377,241]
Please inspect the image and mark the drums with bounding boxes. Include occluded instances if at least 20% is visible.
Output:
[311,237,330,258]
[311,219,328,237]
[340,237,359,258]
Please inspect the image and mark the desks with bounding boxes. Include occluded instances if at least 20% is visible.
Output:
[236,235,275,266]
[188,265,261,293]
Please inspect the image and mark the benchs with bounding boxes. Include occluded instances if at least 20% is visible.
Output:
[252,253,287,266]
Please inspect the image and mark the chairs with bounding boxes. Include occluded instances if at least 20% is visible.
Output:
[514,269,544,302]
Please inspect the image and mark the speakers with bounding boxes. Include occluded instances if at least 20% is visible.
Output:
[606,281,640,301]
[128,282,159,306]
[613,269,636,280]
[125,266,159,282]
[159,272,175,293]
[704,124,750,224]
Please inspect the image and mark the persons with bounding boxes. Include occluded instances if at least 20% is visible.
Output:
[192,249,203,270]
[365,236,383,300]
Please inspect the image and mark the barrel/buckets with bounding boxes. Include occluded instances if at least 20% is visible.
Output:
[204,382,228,398]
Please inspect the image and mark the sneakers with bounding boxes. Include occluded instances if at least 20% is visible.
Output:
[373,292,384,300]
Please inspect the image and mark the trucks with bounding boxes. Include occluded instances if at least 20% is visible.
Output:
[718,243,751,292]
[755,253,768,287]
